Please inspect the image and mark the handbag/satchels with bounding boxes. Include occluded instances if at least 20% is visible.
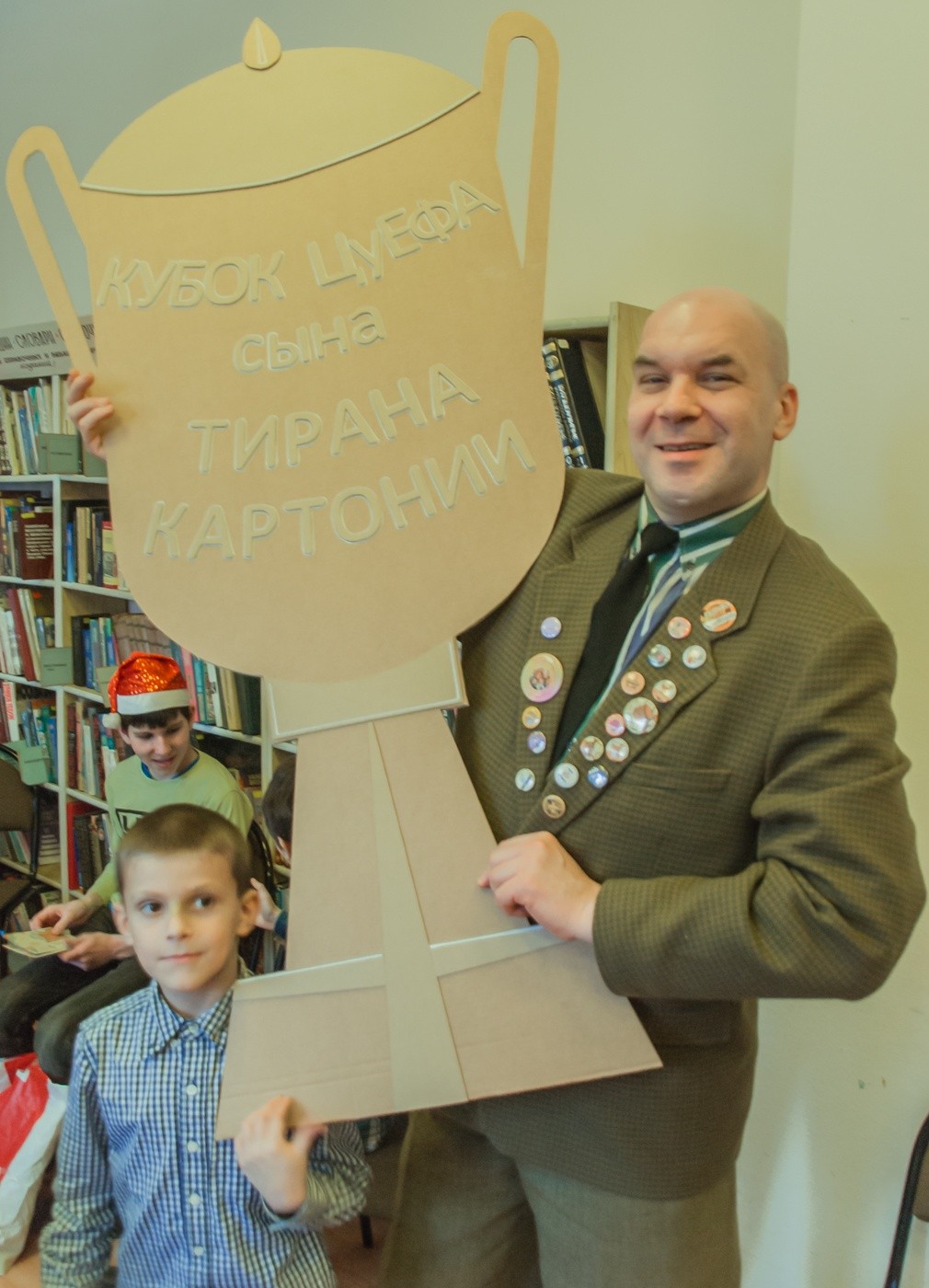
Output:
[0,1050,69,1277]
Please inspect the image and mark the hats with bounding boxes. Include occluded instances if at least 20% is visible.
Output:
[101,651,195,729]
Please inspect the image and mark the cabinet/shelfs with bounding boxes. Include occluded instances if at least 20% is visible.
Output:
[0,301,655,982]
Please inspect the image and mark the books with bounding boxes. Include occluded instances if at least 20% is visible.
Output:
[1,373,108,476]
[547,369,577,471]
[61,498,132,591]
[542,333,606,471]
[0,489,53,581]
[0,609,295,974]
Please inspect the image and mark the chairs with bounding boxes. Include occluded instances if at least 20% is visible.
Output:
[0,758,44,929]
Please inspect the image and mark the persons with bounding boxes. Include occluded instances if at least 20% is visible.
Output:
[0,651,256,1084]
[69,294,928,1287]
[252,757,300,935]
[35,804,369,1287]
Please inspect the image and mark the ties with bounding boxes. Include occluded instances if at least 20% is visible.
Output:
[548,521,681,773]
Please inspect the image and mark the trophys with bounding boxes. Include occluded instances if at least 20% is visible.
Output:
[0,0,662,1140]
